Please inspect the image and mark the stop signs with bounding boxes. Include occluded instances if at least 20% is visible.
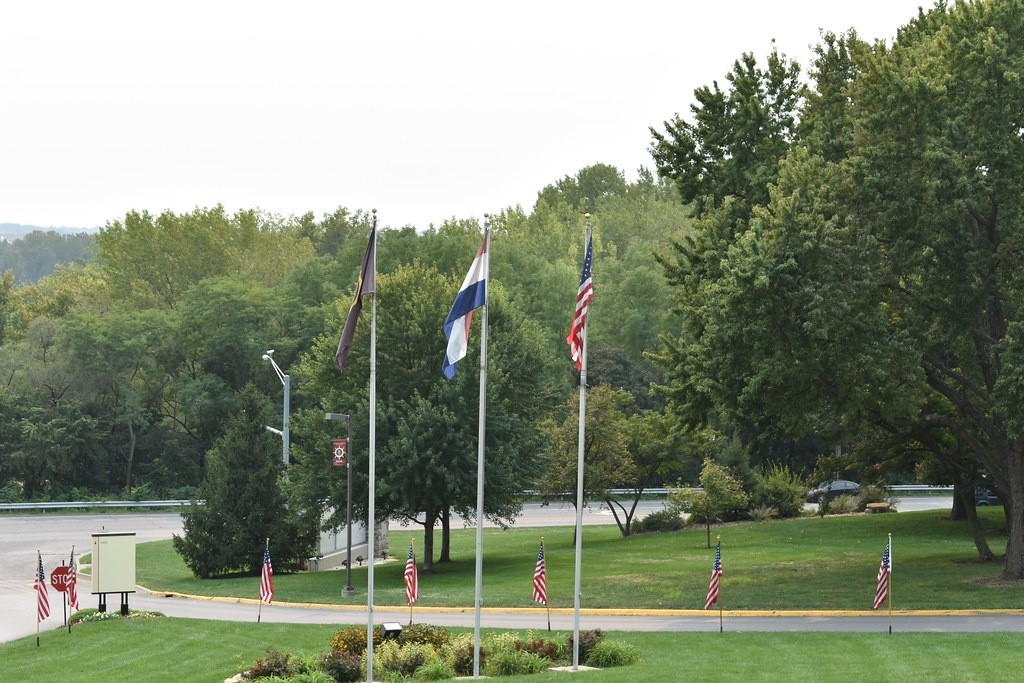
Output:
[51,567,71,593]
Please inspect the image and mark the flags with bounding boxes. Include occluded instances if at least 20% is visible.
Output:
[258,546,274,605]
[532,542,546,605]
[872,538,892,610]
[441,228,490,379]
[335,223,375,372]
[403,542,418,606]
[33,552,51,623]
[331,438,347,466]
[704,543,722,609]
[566,221,594,373]
[65,550,80,612]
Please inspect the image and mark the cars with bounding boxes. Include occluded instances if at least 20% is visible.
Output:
[807,480,861,505]
[976,474,1002,507]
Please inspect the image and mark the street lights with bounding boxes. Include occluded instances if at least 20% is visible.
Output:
[261,347,290,468]
[326,413,355,590]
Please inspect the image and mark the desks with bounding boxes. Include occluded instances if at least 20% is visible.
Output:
[866,503,891,513]
